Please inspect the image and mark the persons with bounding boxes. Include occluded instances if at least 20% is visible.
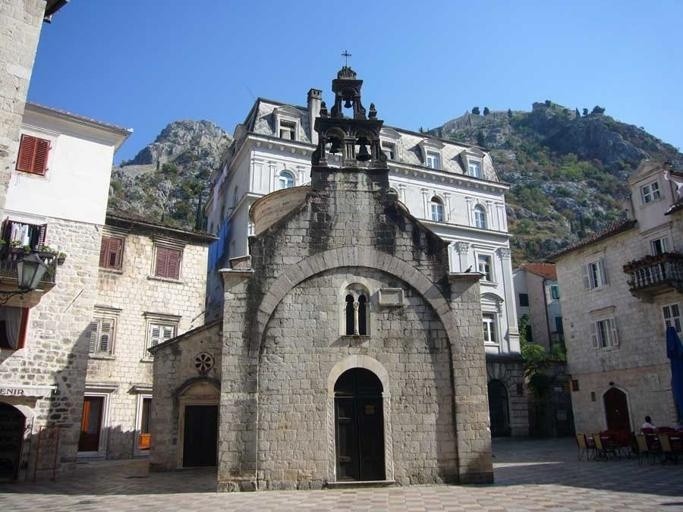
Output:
[642,416,656,429]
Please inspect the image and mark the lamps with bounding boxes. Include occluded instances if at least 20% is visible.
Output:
[0,243,51,306]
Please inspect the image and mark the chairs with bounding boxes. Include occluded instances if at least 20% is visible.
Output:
[575,426,683,466]
